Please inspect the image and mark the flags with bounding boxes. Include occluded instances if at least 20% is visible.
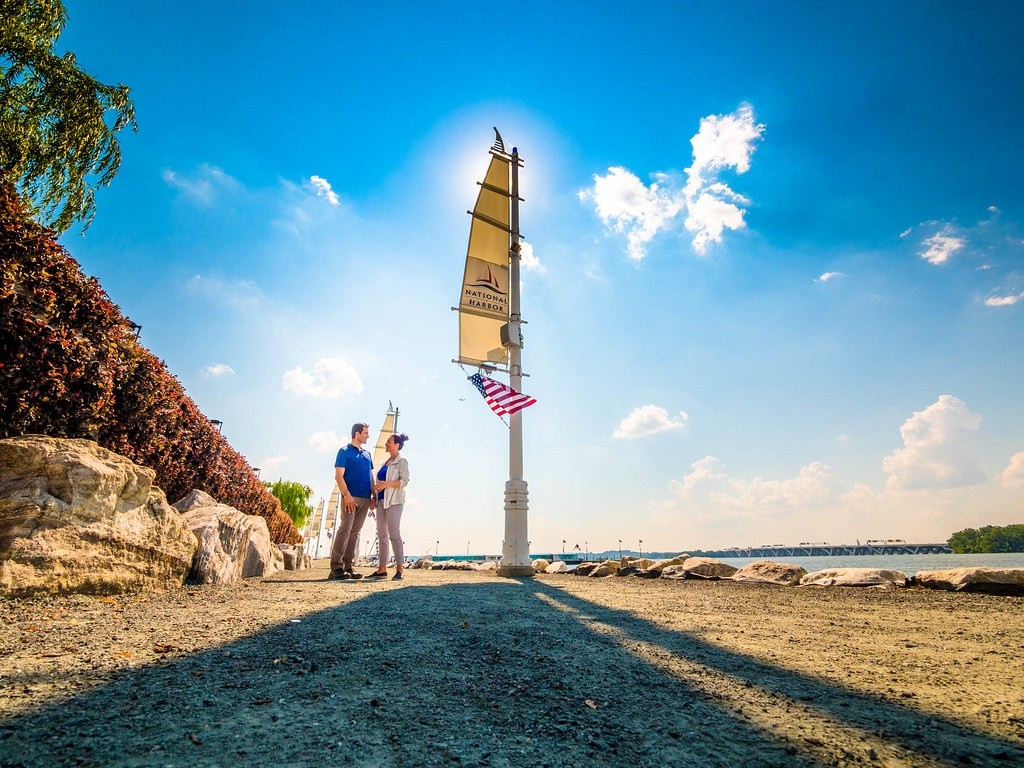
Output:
[470,373,536,416]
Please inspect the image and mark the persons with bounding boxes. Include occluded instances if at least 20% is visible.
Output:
[329,423,410,581]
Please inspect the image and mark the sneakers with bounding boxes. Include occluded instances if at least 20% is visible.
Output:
[392,571,404,580]
[344,568,362,579]
[365,570,387,579]
[328,567,351,580]
